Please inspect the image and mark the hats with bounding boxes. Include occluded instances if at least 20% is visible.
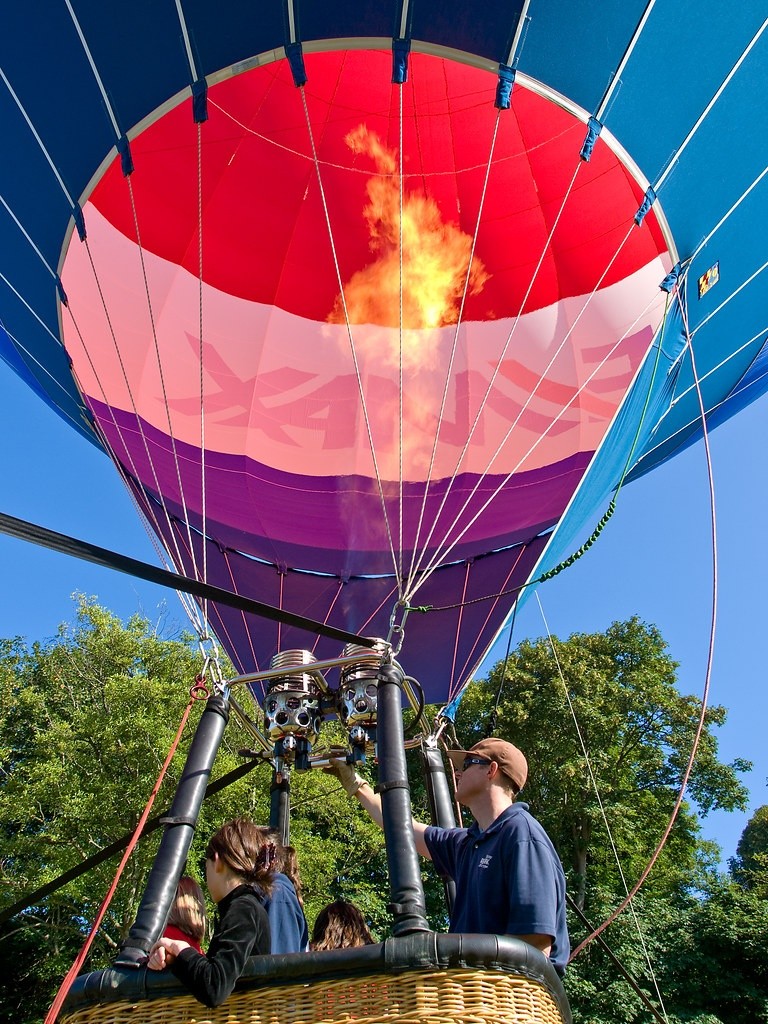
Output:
[447,738,528,792]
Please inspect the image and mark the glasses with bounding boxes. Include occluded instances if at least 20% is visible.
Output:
[462,757,491,772]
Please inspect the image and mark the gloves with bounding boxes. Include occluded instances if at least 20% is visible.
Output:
[322,745,369,798]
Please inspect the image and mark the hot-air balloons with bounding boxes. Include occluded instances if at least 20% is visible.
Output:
[2,1,767,1023]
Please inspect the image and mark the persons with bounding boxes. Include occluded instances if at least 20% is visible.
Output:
[311,902,375,952]
[147,820,309,1007]
[323,737,570,978]
